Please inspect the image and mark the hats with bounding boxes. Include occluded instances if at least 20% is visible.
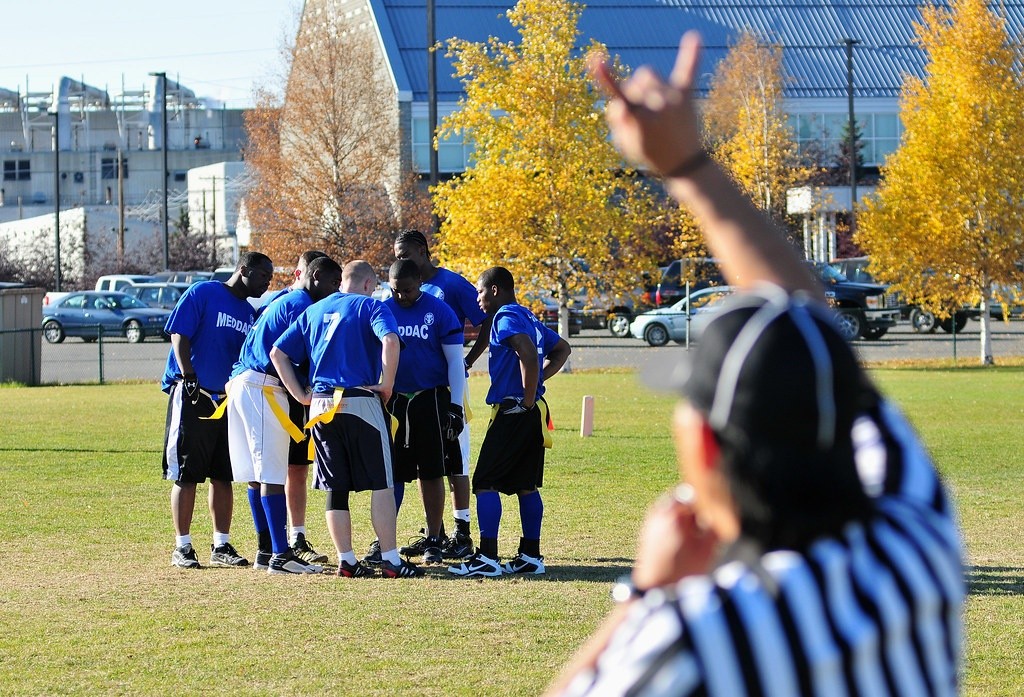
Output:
[638,278,860,462]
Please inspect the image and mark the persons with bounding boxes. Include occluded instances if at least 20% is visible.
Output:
[225,257,342,574]
[160,251,274,569]
[393,229,495,561]
[447,266,571,578]
[257,250,331,563]
[382,258,465,565]
[540,30,965,697]
[269,259,425,579]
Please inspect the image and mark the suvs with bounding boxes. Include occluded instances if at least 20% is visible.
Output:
[828,257,967,334]
[800,260,901,341]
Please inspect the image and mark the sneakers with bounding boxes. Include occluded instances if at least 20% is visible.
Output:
[441,534,474,560]
[267,552,322,574]
[291,532,328,564]
[381,553,427,579]
[209,542,248,566]
[171,543,201,568]
[361,541,382,566]
[400,530,447,557]
[448,550,502,576]
[253,549,271,571]
[420,536,442,568]
[501,554,547,576]
[336,558,376,578]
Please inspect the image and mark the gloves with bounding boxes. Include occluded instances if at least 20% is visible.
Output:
[503,397,535,414]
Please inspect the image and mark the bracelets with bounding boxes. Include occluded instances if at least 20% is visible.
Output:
[661,147,710,193]
[608,578,647,601]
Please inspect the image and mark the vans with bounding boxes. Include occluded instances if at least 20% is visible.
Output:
[636,267,669,305]
[94,275,155,292]
[656,258,728,309]
[563,270,650,338]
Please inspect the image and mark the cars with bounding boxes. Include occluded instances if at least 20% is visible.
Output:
[630,285,736,346]
[42,290,172,343]
[519,292,581,336]
[119,282,190,311]
